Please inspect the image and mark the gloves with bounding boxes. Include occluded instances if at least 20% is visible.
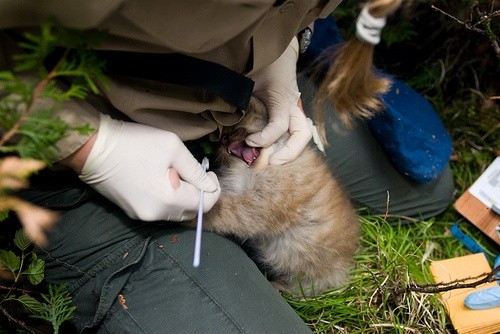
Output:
[77,112,221,221]
[246,36,312,166]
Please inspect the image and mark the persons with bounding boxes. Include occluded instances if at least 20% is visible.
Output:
[0,0,456,334]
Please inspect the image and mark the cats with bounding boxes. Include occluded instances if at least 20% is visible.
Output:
[180,96,360,301]
